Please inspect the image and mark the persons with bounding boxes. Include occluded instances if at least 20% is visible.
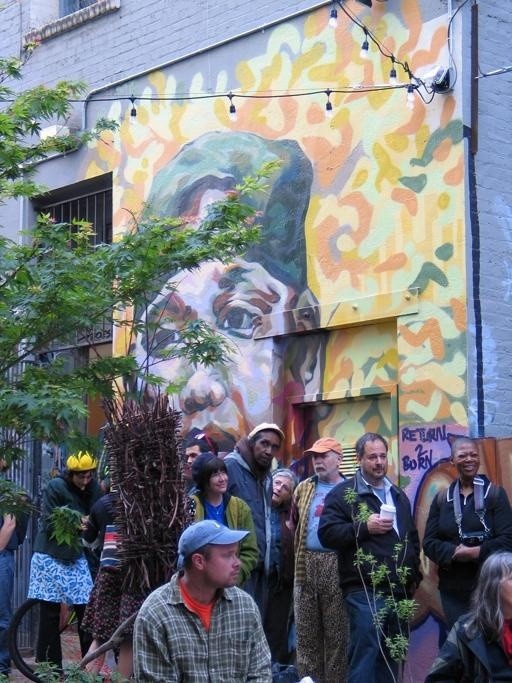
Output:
[0,455,18,683]
[422,438,512,629]
[126,132,322,467]
[425,551,512,683]
[132,519,272,683]
[28,450,196,681]
[186,424,298,666]
[290,438,349,683]
[317,433,422,683]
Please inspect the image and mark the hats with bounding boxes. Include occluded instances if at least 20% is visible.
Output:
[177,519,251,572]
[67,449,98,471]
[204,432,219,455]
[247,421,285,441]
[303,437,342,456]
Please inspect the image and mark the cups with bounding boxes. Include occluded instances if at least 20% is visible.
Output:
[380,504,398,518]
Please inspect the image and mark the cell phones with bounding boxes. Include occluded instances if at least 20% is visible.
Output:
[464,538,473,547]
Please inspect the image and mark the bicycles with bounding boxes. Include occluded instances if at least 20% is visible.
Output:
[9,547,105,683]
